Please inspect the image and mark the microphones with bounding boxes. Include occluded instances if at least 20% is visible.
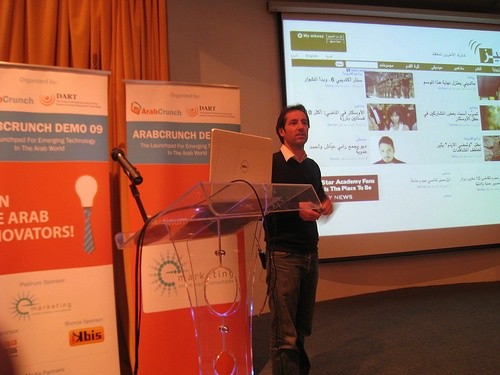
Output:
[110,148,143,185]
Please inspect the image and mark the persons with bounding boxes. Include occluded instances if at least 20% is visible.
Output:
[374,135,405,165]
[365,71,417,131]
[259,102,335,374]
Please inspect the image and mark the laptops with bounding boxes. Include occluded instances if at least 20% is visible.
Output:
[171,129,274,240]
